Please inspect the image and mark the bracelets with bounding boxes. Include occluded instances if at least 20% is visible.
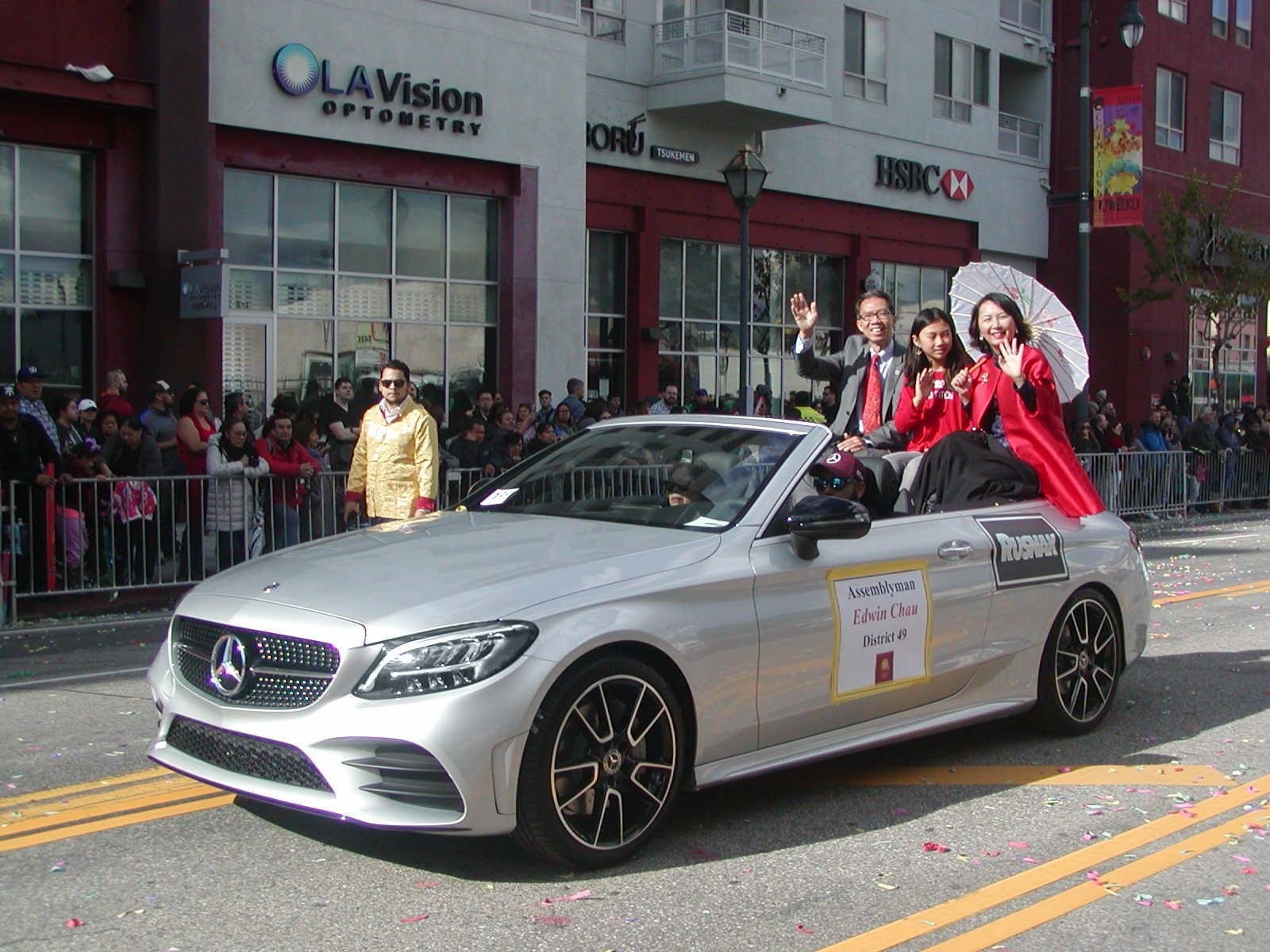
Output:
[349,425,353,432]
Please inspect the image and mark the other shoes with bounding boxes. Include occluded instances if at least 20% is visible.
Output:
[56,561,64,579]
[69,576,98,590]
[1140,512,1159,520]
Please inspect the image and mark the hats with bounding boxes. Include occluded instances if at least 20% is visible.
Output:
[1088,401,1098,411]
[77,398,97,411]
[0,386,20,400]
[1169,380,1177,386]
[17,365,46,382]
[809,451,867,485]
[1096,389,1107,401]
[151,380,170,393]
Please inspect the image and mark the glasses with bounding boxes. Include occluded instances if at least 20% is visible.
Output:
[1105,408,1113,411]
[1083,426,1089,430]
[813,477,859,491]
[857,310,892,321]
[1159,409,1166,412]
[196,399,211,405]
[663,478,693,492]
[380,379,408,388]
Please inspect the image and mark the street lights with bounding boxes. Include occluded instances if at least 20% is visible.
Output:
[715,142,774,415]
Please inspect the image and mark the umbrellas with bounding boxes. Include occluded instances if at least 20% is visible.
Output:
[949,261,1090,404]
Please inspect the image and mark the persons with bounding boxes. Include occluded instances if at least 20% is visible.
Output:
[1066,389,1136,512]
[660,463,713,507]
[344,359,439,526]
[409,378,690,509]
[1215,404,1270,514]
[1122,402,1225,520]
[1177,376,1190,433]
[790,290,906,518]
[907,292,1106,516]
[874,307,971,519]
[1160,380,1179,419]
[692,384,838,489]
[809,451,881,521]
[0,364,369,618]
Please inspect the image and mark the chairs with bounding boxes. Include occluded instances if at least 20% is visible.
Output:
[857,454,910,518]
[695,452,751,497]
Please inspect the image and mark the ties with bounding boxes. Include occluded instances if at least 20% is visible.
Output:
[862,354,882,437]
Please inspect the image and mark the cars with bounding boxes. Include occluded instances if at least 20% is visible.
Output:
[140,414,1155,870]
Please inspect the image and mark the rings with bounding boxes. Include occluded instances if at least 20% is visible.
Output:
[1012,351,1018,355]
